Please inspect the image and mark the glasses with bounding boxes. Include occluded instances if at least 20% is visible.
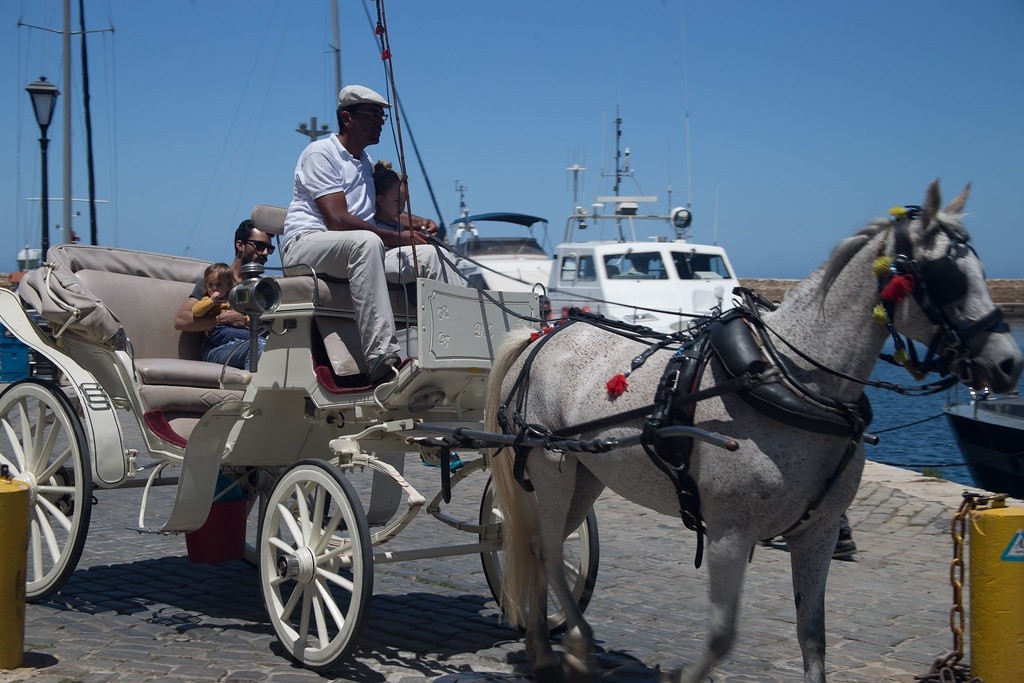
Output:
[350,109,388,120]
[243,239,276,254]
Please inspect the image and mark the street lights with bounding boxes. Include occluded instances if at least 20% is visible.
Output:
[24,75,60,269]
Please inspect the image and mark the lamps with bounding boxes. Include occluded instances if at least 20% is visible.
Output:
[230,263,282,372]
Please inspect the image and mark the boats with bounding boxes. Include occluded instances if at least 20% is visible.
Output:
[941,299,1024,500]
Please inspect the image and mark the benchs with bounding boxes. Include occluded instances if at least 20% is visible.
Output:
[22,243,252,419]
[250,207,416,392]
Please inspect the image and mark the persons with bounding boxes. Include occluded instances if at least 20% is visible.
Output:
[192,263,267,347]
[175,219,276,369]
[281,85,439,383]
[374,159,463,286]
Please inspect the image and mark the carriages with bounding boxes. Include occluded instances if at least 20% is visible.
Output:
[0,176,1023,683]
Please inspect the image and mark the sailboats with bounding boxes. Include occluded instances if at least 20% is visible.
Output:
[2,1,747,346]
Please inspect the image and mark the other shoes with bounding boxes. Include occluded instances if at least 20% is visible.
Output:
[367,352,402,384]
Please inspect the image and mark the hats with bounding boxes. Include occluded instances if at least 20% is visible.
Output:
[338,84,393,110]
